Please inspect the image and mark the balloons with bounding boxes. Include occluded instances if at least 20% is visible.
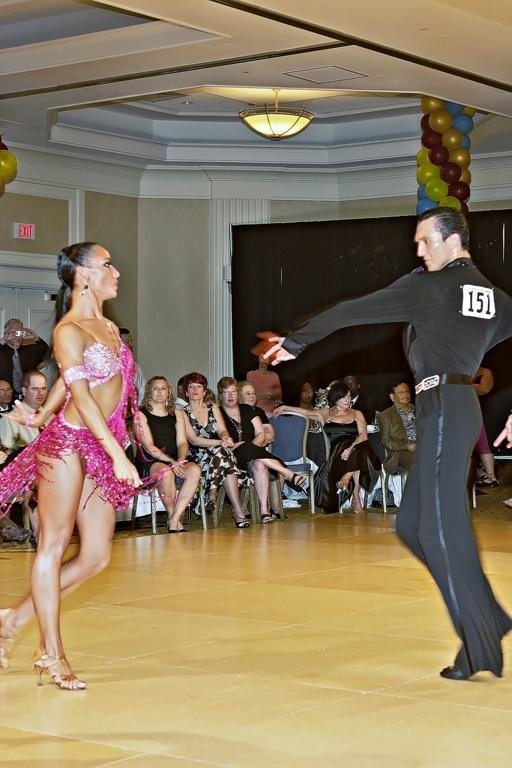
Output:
[415,96,477,217]
[0,135,17,198]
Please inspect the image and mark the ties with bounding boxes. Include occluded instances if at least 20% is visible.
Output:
[12,348,23,393]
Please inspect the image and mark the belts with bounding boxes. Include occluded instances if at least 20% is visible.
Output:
[415,373,472,395]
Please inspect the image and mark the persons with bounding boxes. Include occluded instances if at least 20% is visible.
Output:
[1,316,371,542]
[473,367,512,506]
[377,381,479,513]
[263,205,512,679]
[0,243,145,690]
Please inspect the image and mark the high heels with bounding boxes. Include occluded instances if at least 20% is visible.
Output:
[33,651,87,690]
[206,497,215,517]
[336,482,346,494]
[232,511,251,528]
[0,608,22,670]
[1,523,38,552]
[352,497,364,515]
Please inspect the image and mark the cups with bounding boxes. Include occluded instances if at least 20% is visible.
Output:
[367,424,377,431]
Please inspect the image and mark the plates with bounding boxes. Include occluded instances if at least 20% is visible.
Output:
[367,431,376,433]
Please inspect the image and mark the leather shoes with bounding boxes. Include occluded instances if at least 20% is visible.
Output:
[475,473,502,488]
[166,517,187,533]
[441,664,467,680]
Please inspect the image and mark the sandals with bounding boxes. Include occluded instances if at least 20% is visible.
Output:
[285,472,306,485]
[261,508,288,524]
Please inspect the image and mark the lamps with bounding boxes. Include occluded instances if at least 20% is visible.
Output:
[236,87,316,144]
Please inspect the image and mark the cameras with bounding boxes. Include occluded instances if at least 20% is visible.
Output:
[15,331,26,336]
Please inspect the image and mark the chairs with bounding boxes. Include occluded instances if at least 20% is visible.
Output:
[131,412,408,534]
